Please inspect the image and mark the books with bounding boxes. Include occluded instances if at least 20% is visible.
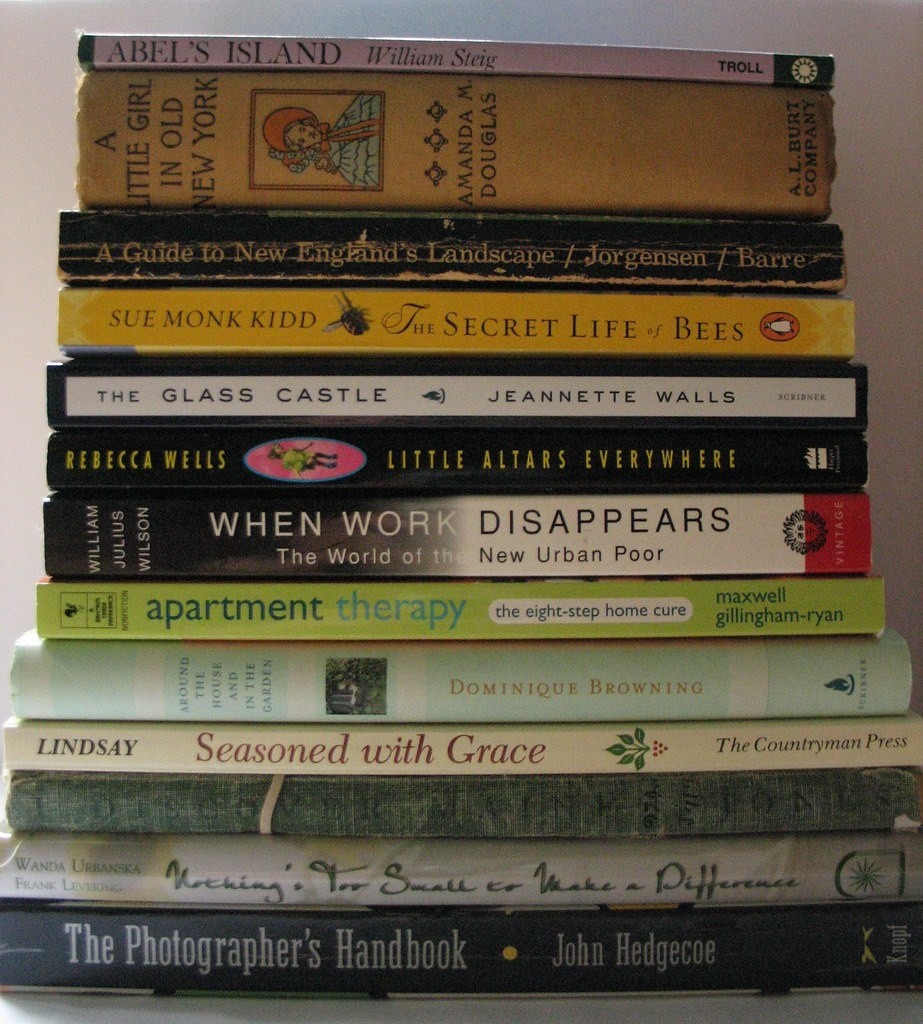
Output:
[1,33,922,990]
